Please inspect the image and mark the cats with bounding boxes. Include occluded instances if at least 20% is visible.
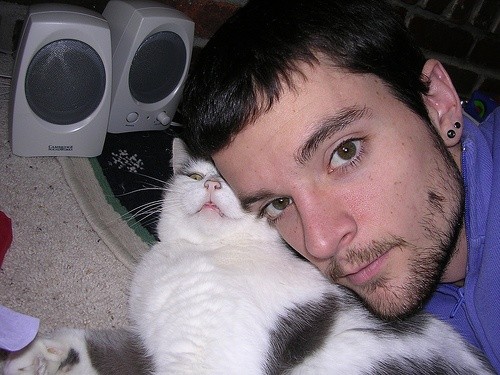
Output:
[0,138,500,375]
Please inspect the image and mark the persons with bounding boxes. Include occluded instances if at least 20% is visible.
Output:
[179,0,500,375]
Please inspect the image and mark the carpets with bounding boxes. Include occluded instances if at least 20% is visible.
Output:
[58,36,210,271]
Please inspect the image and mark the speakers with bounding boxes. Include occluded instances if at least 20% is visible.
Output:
[102,0,195,134]
[8,2,113,157]
